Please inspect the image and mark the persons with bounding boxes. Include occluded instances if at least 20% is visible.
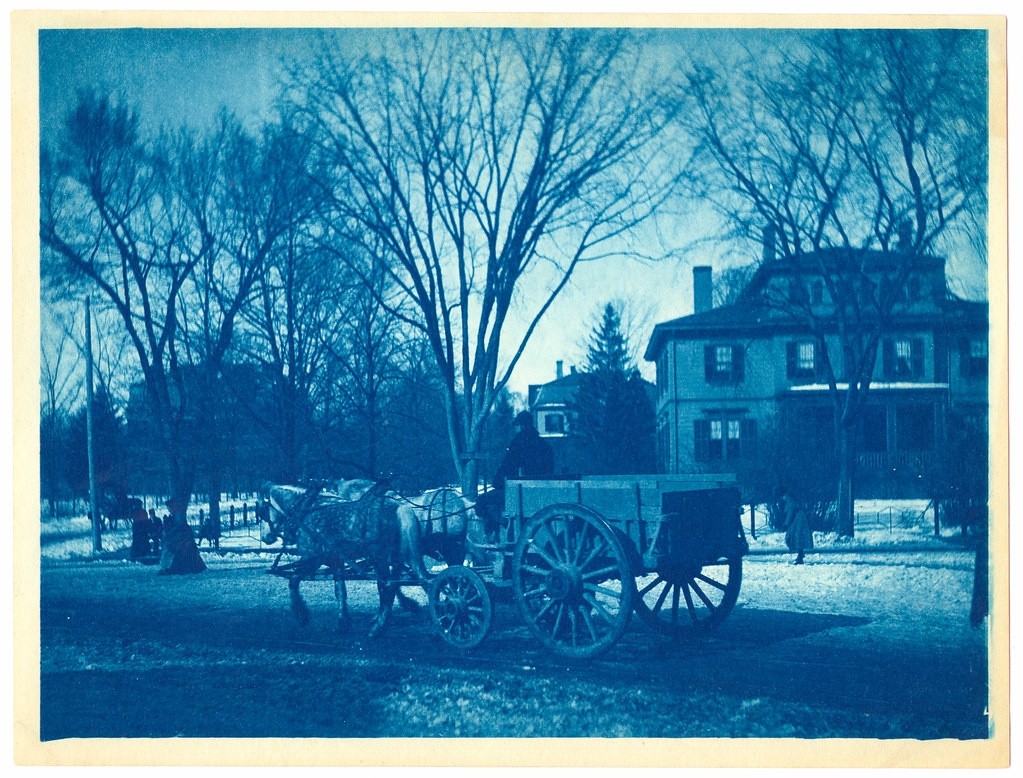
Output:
[776,486,814,564]
[478,410,553,544]
[147,509,164,556]
[196,518,215,547]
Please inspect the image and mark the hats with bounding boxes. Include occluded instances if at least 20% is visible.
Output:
[511,411,533,427]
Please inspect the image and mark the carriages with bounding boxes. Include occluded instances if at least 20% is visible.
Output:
[253,472,749,665]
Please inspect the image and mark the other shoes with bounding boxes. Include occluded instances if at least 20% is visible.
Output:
[794,559,804,565]
[480,530,500,544]
[795,553,806,561]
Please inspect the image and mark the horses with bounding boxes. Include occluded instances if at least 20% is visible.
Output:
[259,479,480,636]
